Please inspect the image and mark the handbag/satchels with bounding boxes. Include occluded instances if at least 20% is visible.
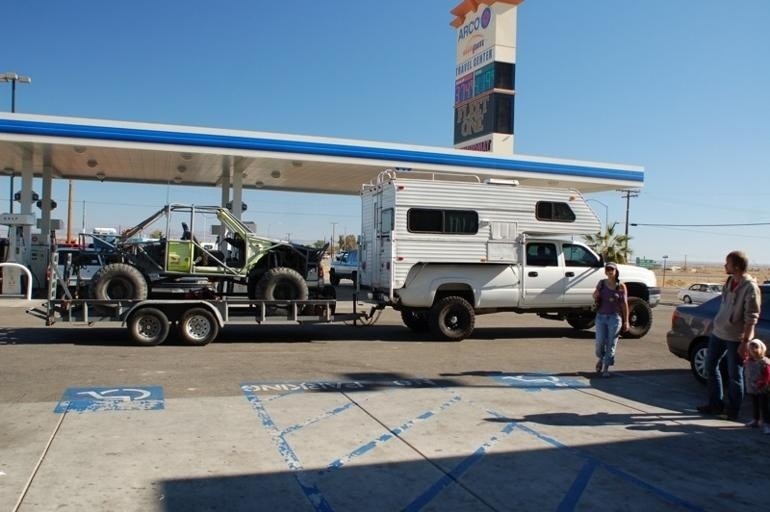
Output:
[590,298,600,312]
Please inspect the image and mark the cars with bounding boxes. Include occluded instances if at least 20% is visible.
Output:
[678,282,723,304]
[666,283,770,385]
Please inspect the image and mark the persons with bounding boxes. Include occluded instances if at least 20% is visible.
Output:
[537,246,548,256]
[736,336,770,435]
[590,261,631,376]
[696,251,762,422]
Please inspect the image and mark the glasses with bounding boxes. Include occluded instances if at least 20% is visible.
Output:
[606,268,615,272]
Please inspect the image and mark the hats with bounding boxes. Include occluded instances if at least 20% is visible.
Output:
[605,262,617,269]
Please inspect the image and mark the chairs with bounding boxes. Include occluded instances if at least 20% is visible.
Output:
[181,222,224,261]
[537,246,549,261]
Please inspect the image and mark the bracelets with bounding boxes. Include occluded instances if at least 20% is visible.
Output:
[742,338,751,343]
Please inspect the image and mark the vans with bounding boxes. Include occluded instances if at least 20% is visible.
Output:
[356,169,663,340]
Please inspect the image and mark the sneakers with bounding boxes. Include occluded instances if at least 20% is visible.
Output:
[696,405,725,413]
[596,360,610,377]
[746,419,770,434]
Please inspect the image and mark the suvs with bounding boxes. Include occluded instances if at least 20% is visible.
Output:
[329,249,359,286]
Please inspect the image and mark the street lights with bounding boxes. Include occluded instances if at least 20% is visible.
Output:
[0,72,32,214]
